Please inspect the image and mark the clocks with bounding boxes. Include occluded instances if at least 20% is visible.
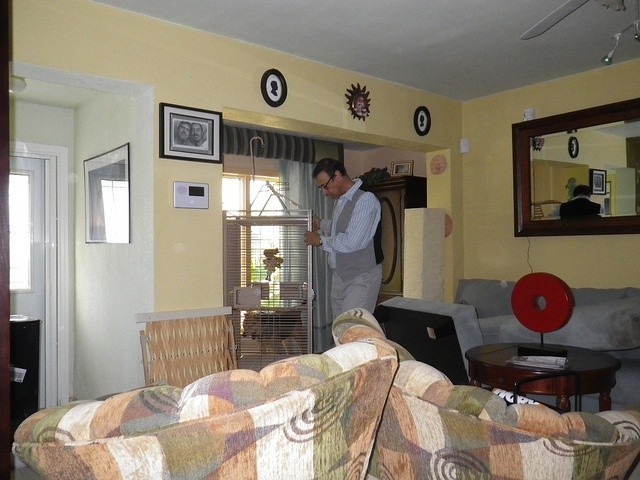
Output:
[568,137,579,157]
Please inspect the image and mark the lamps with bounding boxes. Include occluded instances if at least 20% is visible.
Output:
[9,76,26,95]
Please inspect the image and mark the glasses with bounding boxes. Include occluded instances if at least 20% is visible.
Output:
[318,175,335,191]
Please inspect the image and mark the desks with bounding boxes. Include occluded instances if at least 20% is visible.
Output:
[465,342,620,412]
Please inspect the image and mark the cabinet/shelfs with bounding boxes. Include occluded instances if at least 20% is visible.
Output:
[361,177,427,305]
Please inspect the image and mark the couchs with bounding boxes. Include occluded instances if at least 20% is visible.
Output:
[334,308,640,480]
[11,338,398,479]
[374,279,640,412]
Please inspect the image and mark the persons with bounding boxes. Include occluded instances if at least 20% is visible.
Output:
[190,123,209,150]
[304,157,384,322]
[552,186,606,218]
[174,121,192,146]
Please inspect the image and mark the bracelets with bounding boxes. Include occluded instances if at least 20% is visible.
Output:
[319,238,323,246]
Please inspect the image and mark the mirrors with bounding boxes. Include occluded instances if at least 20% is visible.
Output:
[511,99,639,236]
[83,142,132,245]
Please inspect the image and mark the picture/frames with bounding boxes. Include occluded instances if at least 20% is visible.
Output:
[590,169,606,195]
[391,160,414,177]
[159,102,224,164]
[413,105,431,137]
[260,68,287,109]
